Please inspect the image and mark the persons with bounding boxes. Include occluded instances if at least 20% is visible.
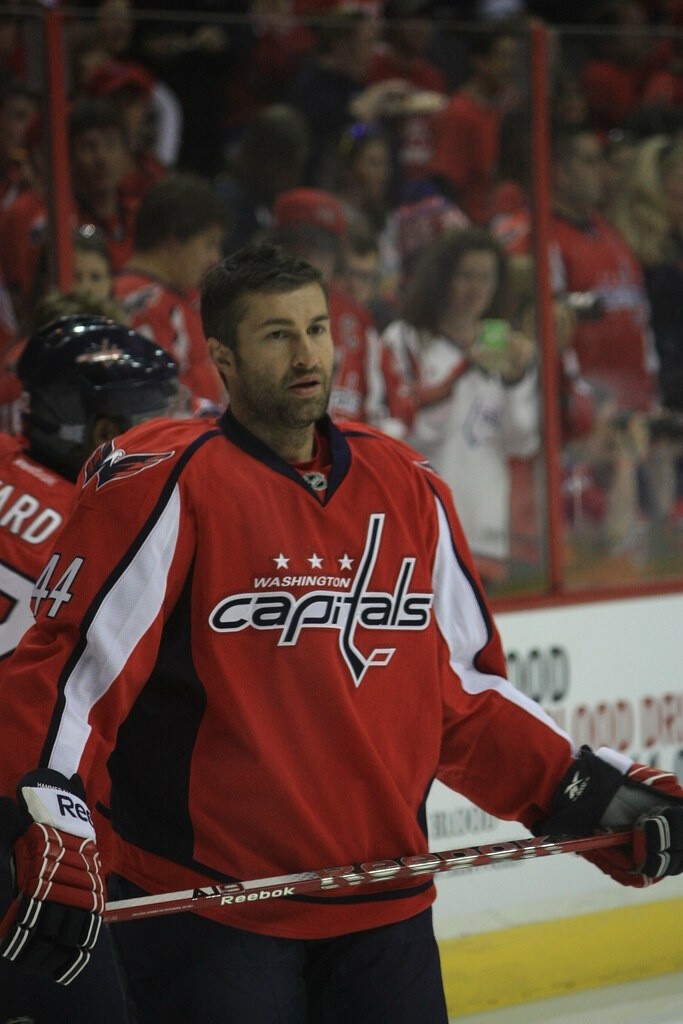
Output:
[1,305,198,649]
[2,0,683,590]
[1,249,683,1016]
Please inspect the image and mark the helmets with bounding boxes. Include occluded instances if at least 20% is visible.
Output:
[17,314,180,414]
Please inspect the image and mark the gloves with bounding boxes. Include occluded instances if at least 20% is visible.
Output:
[528,744,682,888]
[0,768,111,1023]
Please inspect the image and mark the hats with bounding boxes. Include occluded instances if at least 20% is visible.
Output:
[276,191,346,236]
